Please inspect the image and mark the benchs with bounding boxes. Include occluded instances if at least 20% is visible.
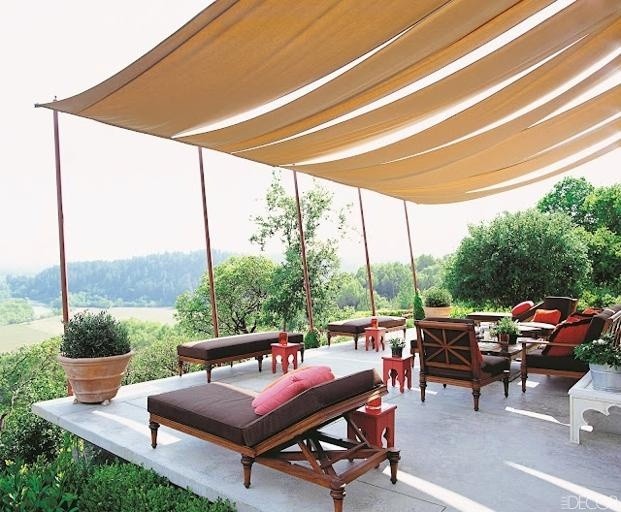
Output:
[177,331,304,383]
[327,316,407,350]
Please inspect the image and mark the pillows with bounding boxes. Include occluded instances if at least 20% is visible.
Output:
[250,365,335,416]
[542,304,621,357]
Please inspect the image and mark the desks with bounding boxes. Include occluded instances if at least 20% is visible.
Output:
[269,343,297,374]
[347,404,398,468]
[568,370,621,445]
[382,354,414,394]
[364,327,386,352]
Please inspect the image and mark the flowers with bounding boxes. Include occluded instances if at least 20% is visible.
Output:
[572,334,621,370]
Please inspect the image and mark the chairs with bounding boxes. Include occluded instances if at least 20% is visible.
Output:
[148,368,401,512]
[414,295,578,411]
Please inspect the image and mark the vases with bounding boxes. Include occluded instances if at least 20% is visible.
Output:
[589,362,621,392]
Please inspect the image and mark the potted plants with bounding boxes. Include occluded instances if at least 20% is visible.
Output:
[385,338,406,357]
[421,286,453,318]
[57,307,133,404]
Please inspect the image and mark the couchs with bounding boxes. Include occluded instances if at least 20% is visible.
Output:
[518,310,621,394]
